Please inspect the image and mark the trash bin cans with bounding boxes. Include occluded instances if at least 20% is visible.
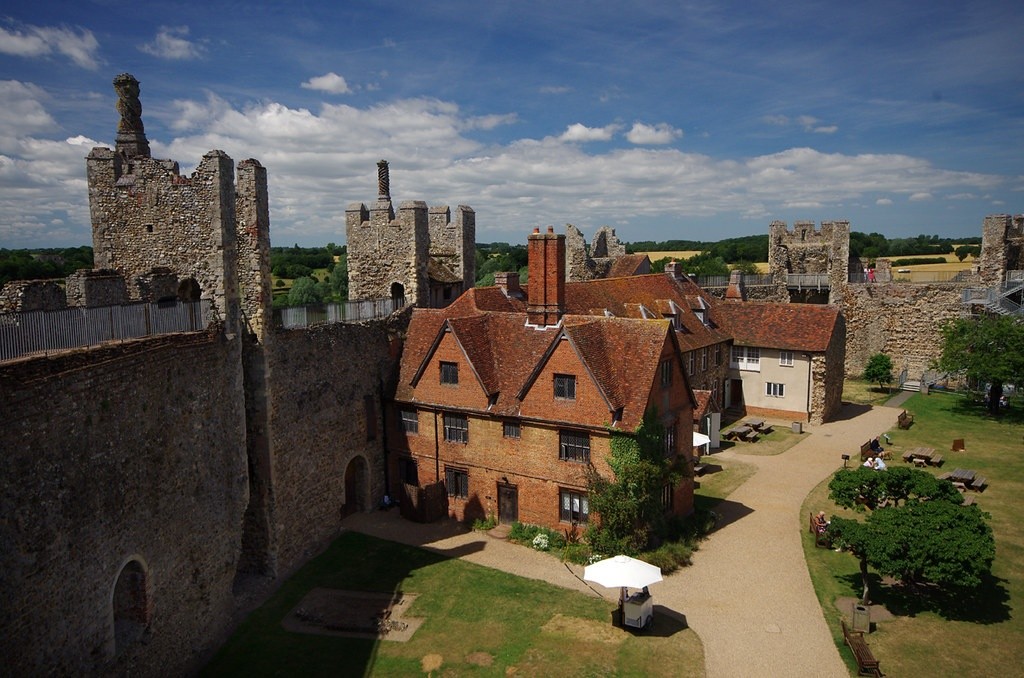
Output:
[792,421,802,434]
[852,603,870,633]
[920,382,929,395]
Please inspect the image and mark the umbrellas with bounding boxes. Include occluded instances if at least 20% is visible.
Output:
[693,432,711,447]
[583,554,663,603]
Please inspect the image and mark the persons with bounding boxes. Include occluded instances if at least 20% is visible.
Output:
[862,436,887,470]
[815,511,828,534]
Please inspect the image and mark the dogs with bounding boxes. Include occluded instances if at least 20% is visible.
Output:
[878,451,894,462]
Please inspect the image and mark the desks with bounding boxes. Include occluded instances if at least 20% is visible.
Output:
[950,468,977,489]
[913,458,925,468]
[729,426,752,442]
[912,446,935,459]
[745,418,768,431]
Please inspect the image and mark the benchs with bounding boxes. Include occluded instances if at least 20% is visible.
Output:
[952,481,965,488]
[937,471,952,480]
[809,511,833,549]
[897,410,914,430]
[758,424,773,434]
[721,428,732,440]
[841,619,887,678]
[970,476,986,494]
[959,495,975,508]
[745,431,759,444]
[901,450,913,462]
[860,438,876,461]
[694,462,710,473]
[930,454,943,466]
